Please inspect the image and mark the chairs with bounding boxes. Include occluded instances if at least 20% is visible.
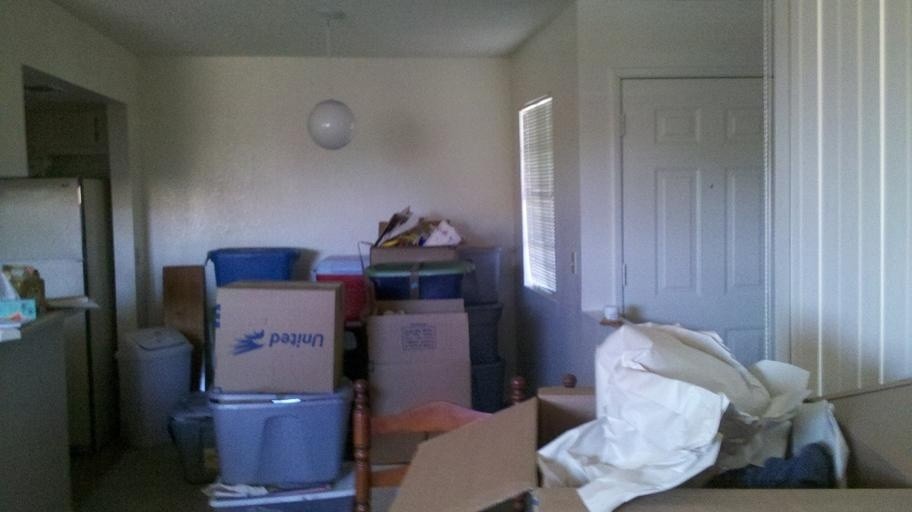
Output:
[355,376,526,512]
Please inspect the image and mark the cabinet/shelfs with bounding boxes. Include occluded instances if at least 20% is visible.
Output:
[0,309,76,512]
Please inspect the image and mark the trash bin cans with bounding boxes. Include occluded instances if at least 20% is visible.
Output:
[114,326,194,435]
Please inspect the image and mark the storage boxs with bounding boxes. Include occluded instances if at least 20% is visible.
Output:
[390,379,912,511]
[365,238,504,466]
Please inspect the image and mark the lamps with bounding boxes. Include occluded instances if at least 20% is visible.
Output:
[307,18,357,149]
